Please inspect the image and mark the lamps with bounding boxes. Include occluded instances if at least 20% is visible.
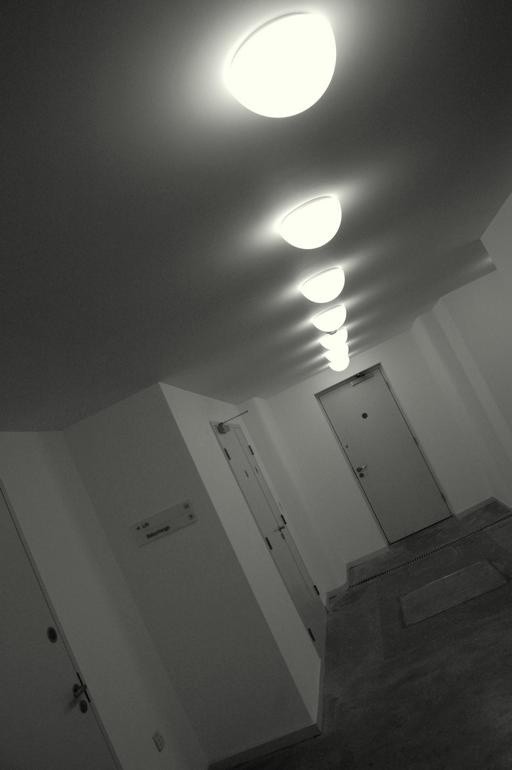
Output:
[297,265,351,373]
[275,194,343,250]
[223,8,339,120]
